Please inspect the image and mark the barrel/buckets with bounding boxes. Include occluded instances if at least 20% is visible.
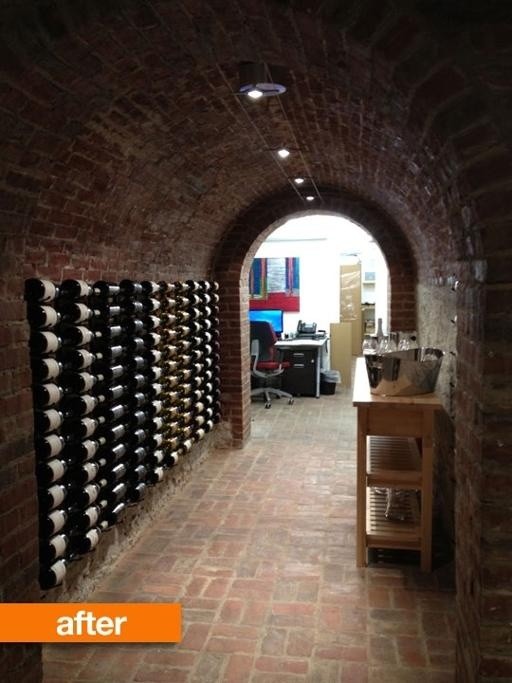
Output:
[363,348,445,396]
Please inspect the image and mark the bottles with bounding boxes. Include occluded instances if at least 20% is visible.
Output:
[361,331,418,354]
[374,486,390,495]
[24,277,222,590]
[385,487,405,521]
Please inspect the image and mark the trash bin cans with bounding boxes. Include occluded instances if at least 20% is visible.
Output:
[321,370,339,395]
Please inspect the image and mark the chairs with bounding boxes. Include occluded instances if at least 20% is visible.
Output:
[249,320,294,408]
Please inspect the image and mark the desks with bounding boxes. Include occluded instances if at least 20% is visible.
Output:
[352,357,441,573]
[274,336,327,398]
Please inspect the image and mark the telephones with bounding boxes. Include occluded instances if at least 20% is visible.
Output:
[297,320,317,334]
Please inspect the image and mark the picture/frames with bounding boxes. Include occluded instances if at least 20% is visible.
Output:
[365,271,376,280]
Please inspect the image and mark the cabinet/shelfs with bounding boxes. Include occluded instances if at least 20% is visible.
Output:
[328,264,363,388]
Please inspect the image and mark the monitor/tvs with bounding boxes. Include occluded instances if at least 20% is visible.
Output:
[249,309,284,333]
